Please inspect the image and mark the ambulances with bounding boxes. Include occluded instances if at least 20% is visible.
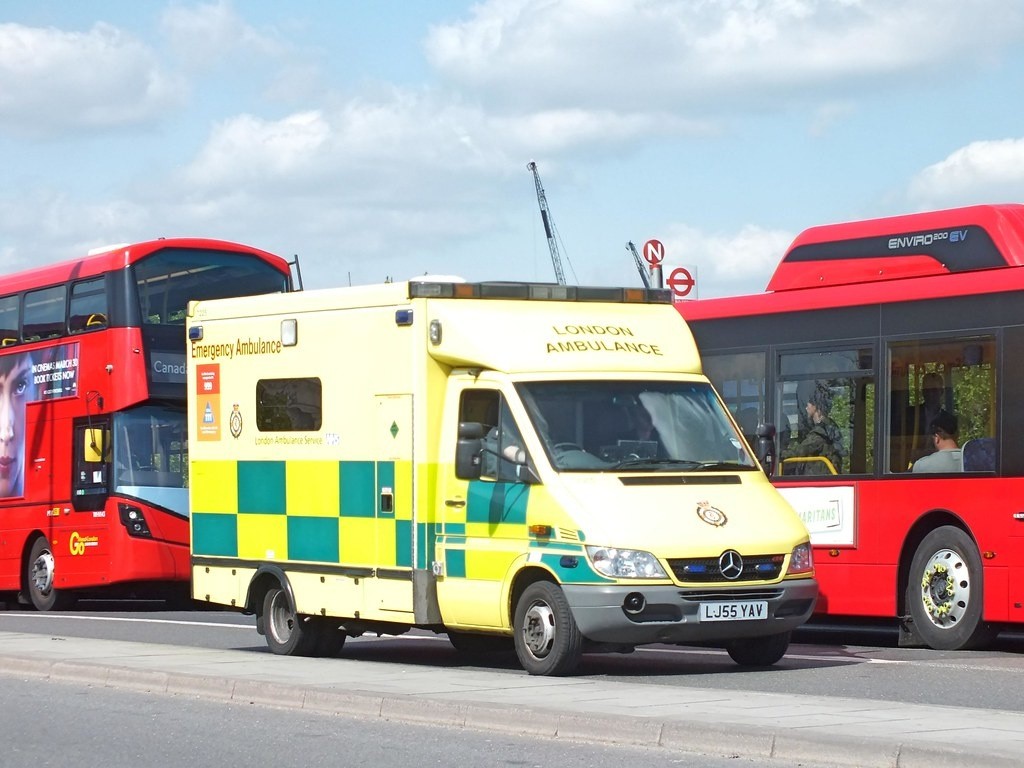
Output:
[186,274,818,680]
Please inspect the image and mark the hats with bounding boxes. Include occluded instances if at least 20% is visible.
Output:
[738,407,757,417]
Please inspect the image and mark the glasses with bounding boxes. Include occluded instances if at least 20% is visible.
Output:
[922,387,937,392]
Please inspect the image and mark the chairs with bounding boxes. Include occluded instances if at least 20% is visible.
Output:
[86,312,108,331]
[962,438,996,471]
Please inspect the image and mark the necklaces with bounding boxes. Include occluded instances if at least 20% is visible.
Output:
[817,416,824,422]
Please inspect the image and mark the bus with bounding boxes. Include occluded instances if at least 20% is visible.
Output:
[639,204,1024,651]
[1,237,295,615]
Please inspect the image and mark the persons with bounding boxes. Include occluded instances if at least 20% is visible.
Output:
[0,351,39,497]
[740,406,774,472]
[483,399,525,477]
[621,403,673,458]
[913,415,963,472]
[906,373,946,460]
[782,395,843,474]
